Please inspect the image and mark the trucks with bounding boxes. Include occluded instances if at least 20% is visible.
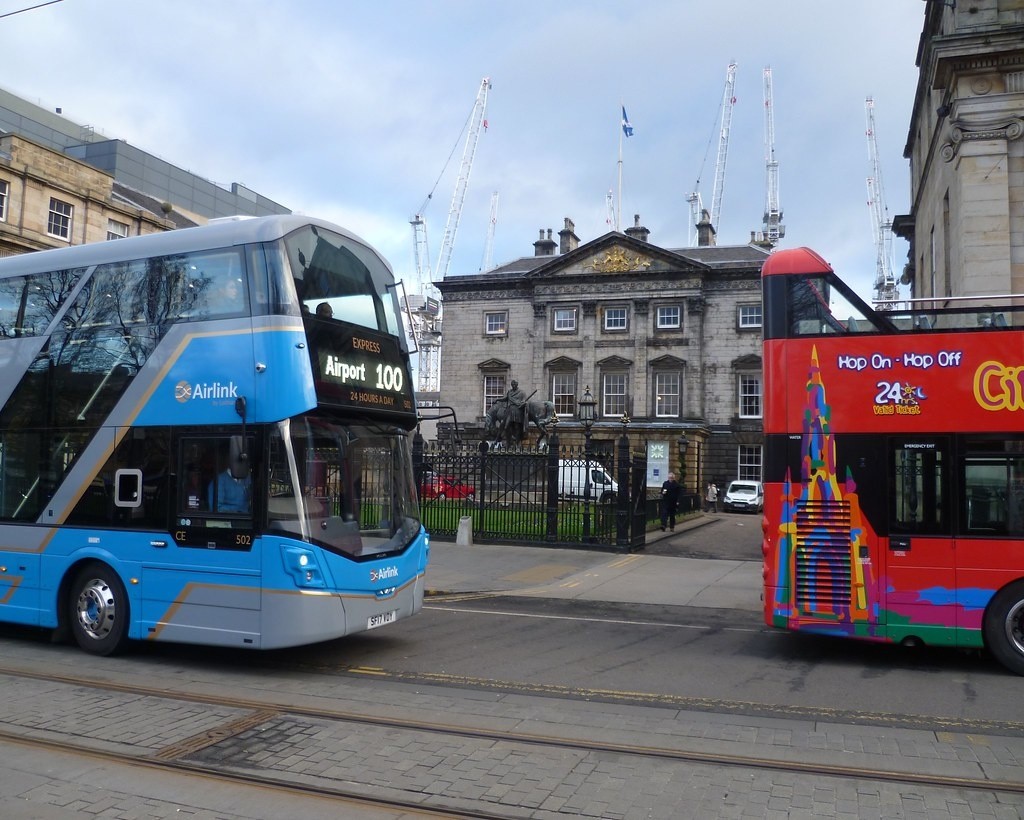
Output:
[558,459,631,503]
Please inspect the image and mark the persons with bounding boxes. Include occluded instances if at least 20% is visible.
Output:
[208,448,252,514]
[217,276,237,299]
[316,302,333,317]
[703,481,718,513]
[659,472,680,531]
[505,380,528,446]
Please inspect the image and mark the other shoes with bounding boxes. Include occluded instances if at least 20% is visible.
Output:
[670,529,674,532]
[660,525,666,532]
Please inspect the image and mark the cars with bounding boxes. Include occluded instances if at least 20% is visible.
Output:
[723,480,763,514]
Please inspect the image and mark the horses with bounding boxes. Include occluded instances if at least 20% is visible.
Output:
[485,401,553,451]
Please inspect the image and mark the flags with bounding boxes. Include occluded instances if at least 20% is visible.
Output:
[622,107,633,137]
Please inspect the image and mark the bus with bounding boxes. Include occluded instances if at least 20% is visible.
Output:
[0,214,430,657]
[761,246,1024,677]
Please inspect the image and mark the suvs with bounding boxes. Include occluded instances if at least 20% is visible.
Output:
[420,471,476,502]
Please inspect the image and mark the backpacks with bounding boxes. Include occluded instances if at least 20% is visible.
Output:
[711,487,721,498]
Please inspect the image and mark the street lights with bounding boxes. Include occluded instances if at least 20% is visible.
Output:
[677,430,689,489]
[578,385,598,543]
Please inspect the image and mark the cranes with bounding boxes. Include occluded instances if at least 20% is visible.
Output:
[685,63,738,248]
[866,95,900,311]
[762,65,785,251]
[400,76,493,407]
